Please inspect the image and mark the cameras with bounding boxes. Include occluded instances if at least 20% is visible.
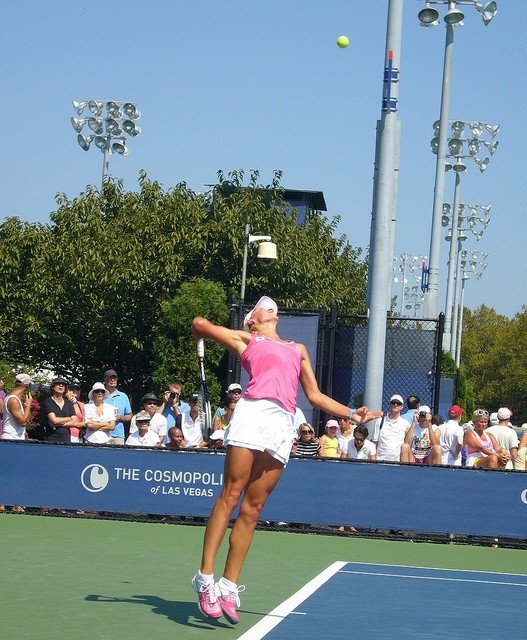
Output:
[419,411,427,418]
[170,392,175,399]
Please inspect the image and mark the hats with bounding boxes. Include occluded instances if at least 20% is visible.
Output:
[326,419,338,428]
[104,369,117,378]
[473,409,489,421]
[225,383,242,394]
[210,430,225,441]
[390,394,404,405]
[88,382,110,400]
[50,378,68,390]
[135,411,151,421]
[15,373,34,386]
[448,405,465,420]
[244,295,278,332]
[490,413,499,425]
[189,393,202,402]
[70,382,80,389]
[141,393,162,402]
[497,407,513,419]
[418,405,431,414]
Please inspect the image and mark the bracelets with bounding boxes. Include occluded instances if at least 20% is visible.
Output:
[350,408,356,421]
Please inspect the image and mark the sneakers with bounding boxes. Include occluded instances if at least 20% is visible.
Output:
[191,573,223,619]
[214,578,246,624]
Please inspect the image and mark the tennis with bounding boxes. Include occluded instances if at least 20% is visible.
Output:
[337,35,349,48]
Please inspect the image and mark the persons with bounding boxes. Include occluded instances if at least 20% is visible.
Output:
[212,382,243,423]
[441,406,465,466]
[0,379,6,439]
[261,408,306,527]
[166,427,187,450]
[156,382,192,448]
[211,398,238,450]
[338,425,377,532]
[507,422,513,428]
[400,405,441,465]
[400,396,427,432]
[337,406,353,437]
[490,412,500,427]
[124,411,162,448]
[371,395,412,535]
[191,296,383,624]
[128,391,168,450]
[65,383,86,443]
[431,414,448,465]
[175,393,212,523]
[463,409,510,470]
[39,378,78,515]
[487,407,519,470]
[76,382,116,516]
[517,422,527,471]
[89,370,133,445]
[311,420,348,531]
[291,422,322,527]
[1,373,35,514]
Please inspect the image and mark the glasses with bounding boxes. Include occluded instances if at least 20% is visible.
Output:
[226,407,234,411]
[391,402,402,405]
[232,389,241,394]
[475,410,489,417]
[94,390,105,393]
[300,430,313,435]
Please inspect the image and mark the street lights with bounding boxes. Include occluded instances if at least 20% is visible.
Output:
[71,99,141,194]
[430,120,499,353]
[234,234,279,383]
[403,285,424,329]
[392,252,418,317]
[417,0,496,331]
[447,250,488,369]
[441,203,490,363]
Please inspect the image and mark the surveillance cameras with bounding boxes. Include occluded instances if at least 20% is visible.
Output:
[255,241,279,267]
